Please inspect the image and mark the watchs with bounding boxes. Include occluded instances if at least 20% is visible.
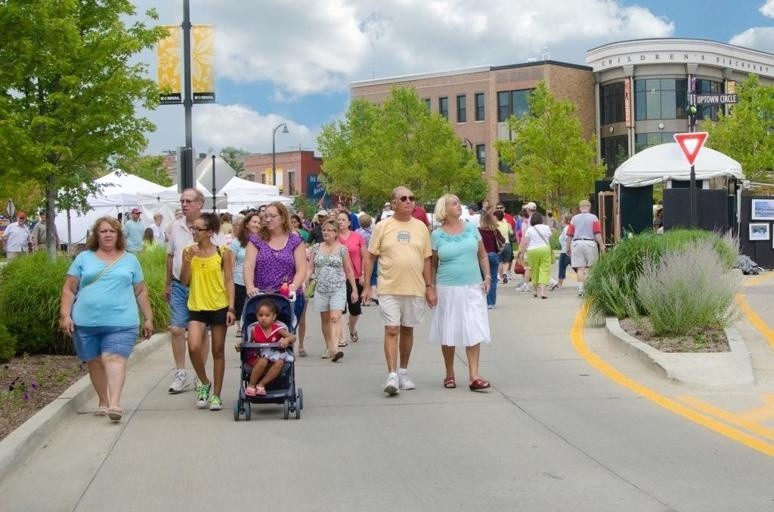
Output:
[426,284,435,287]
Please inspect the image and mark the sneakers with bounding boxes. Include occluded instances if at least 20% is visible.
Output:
[193,374,223,410]
[384,373,415,396]
[169,370,191,392]
[516,285,530,292]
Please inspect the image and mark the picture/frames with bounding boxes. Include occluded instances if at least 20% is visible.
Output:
[748,197,774,223]
[748,224,771,244]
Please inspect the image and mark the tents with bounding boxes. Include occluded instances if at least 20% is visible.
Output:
[138,174,281,235]
[55,167,180,247]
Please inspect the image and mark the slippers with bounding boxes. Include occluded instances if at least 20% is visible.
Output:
[322,331,358,362]
[245,385,266,396]
[470,379,490,390]
[444,378,455,388]
[96,407,122,420]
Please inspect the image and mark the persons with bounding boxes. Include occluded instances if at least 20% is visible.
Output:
[1,208,61,262]
[217,199,577,362]
[180,212,235,410]
[361,185,436,394]
[59,215,154,420]
[567,199,605,297]
[164,188,225,393]
[654,208,664,230]
[235,299,296,396]
[431,193,491,390]
[118,207,183,254]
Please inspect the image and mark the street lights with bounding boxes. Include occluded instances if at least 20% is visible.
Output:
[271,122,288,185]
[685,106,699,229]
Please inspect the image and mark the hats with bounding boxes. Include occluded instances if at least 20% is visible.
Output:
[132,208,142,213]
[579,200,591,207]
[317,210,328,216]
[525,202,536,210]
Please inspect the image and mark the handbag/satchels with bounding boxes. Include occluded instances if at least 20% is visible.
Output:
[305,280,316,298]
[495,239,504,251]
[514,261,525,274]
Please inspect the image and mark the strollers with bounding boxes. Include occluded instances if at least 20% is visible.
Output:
[233,288,302,421]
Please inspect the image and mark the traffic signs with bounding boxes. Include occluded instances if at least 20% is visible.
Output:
[696,92,738,105]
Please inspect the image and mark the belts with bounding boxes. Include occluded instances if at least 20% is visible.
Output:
[573,238,596,241]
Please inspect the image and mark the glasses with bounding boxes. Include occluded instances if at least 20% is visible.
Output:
[401,196,415,201]
[190,226,208,233]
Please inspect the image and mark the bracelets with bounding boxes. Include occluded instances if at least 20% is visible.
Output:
[228,307,235,314]
[184,255,191,262]
[361,271,364,274]
[289,284,298,290]
[484,275,491,279]
